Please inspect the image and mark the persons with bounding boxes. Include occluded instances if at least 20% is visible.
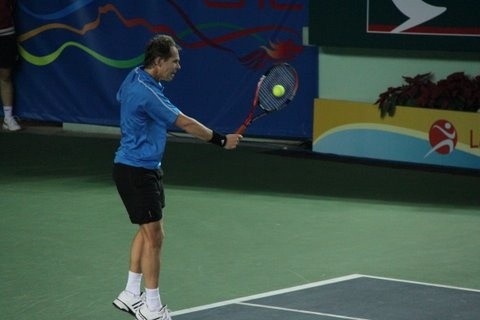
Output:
[112,35,243,320]
[0,1,20,131]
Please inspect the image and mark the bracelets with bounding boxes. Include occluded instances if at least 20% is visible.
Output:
[207,128,227,148]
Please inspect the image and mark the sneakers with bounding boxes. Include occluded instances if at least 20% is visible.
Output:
[135,302,173,320]
[2,115,21,131]
[113,290,146,314]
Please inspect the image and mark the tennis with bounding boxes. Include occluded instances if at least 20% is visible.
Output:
[273,84,285,97]
